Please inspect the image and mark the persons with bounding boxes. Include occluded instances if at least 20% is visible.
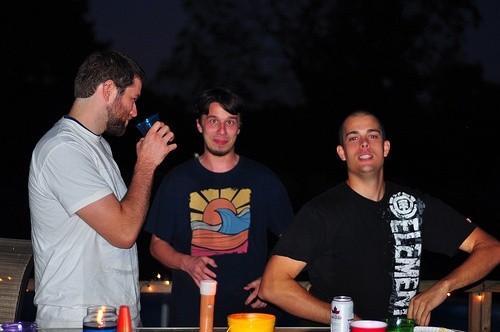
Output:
[144,83,295,326]
[27,48,177,332]
[257,105,500,327]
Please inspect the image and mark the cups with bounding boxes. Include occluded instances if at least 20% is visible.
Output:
[135,114,170,145]
[385,318,415,332]
[350,320,387,332]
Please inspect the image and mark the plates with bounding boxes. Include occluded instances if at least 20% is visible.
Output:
[413,326,454,332]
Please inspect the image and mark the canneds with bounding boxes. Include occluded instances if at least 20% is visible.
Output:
[82,305,118,332]
[330,295,354,332]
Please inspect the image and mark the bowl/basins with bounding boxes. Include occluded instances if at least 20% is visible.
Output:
[227,313,276,332]
